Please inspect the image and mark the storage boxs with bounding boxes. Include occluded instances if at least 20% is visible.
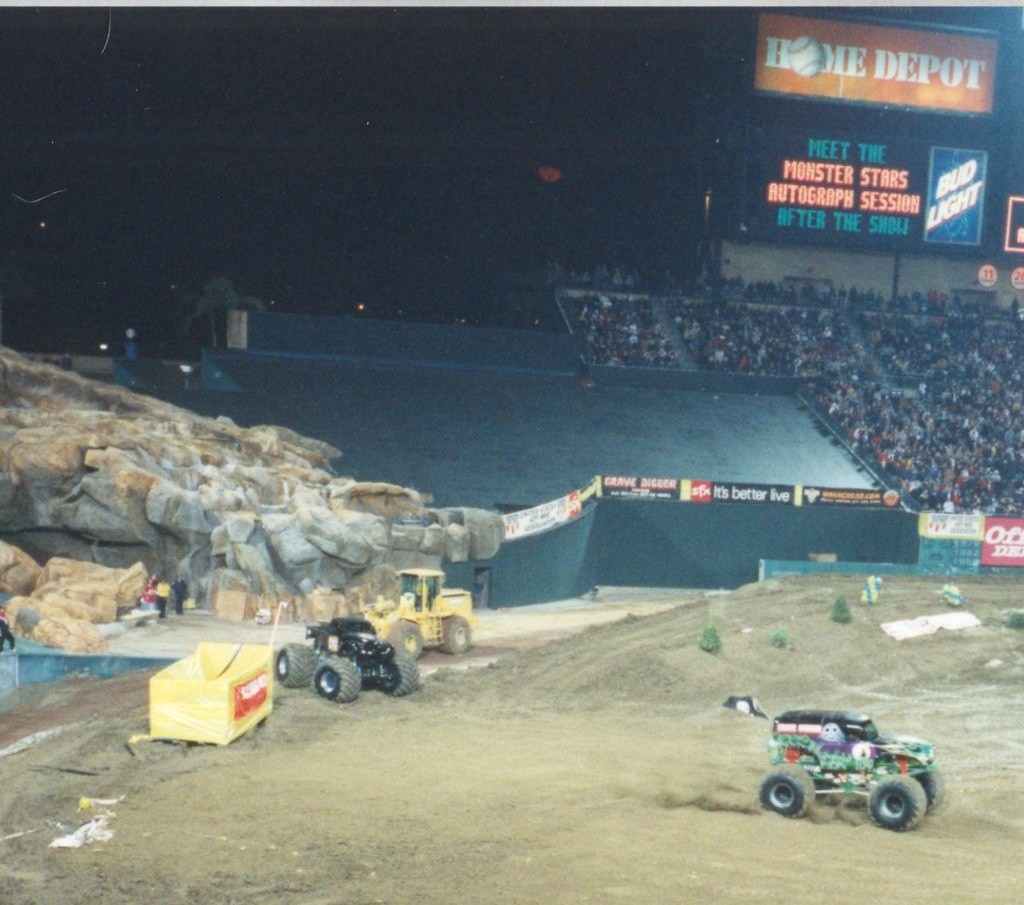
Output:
[147,643,273,745]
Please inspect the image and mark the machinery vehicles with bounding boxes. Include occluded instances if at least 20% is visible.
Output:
[363,567,479,660]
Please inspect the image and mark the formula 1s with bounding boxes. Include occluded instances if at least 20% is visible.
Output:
[759,708,943,833]
[277,617,420,703]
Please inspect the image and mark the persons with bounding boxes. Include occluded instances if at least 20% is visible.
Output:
[669,302,872,375]
[0,606,15,654]
[736,273,1024,517]
[574,293,677,368]
[567,263,647,293]
[144,574,187,617]
